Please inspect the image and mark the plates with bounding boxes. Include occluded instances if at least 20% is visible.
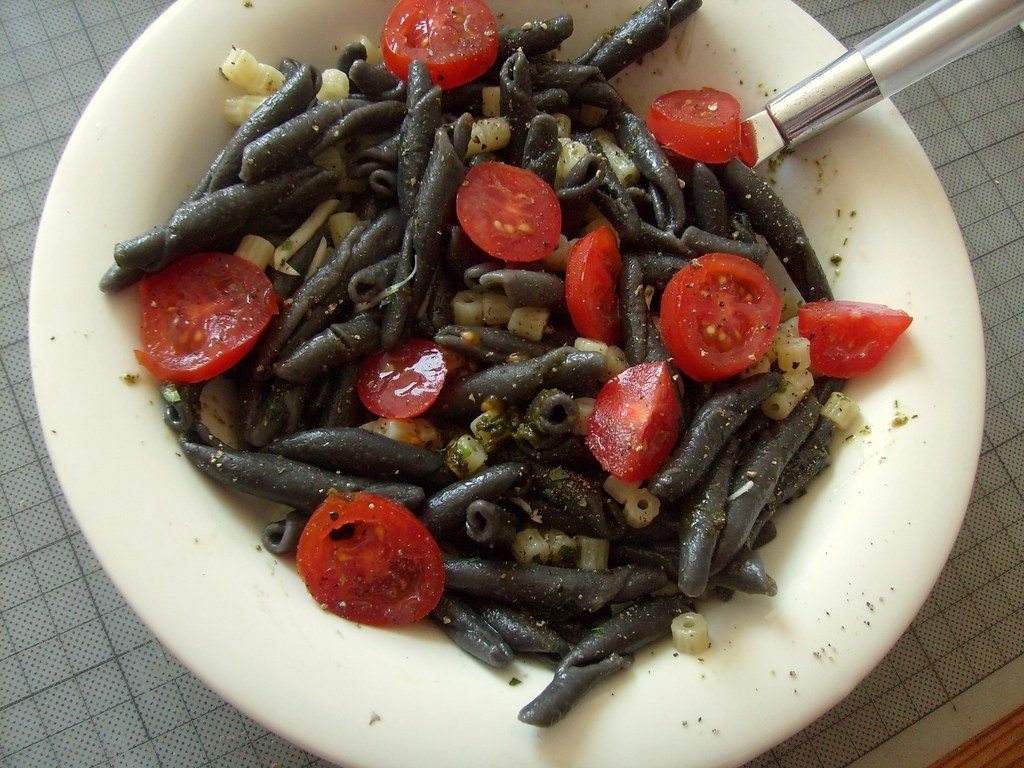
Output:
[28,0,987,768]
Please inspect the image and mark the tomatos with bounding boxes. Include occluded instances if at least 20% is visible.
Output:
[132,0,913,626]
